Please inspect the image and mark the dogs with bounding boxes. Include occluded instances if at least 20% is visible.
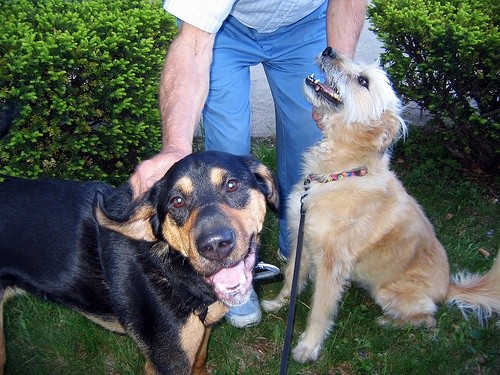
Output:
[261,46,500,364]
[0,98,285,375]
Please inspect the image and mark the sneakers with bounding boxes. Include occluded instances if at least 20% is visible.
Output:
[223,283,262,328]
[252,261,284,284]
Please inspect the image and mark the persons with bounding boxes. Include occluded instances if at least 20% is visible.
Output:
[126,1,399,326]
